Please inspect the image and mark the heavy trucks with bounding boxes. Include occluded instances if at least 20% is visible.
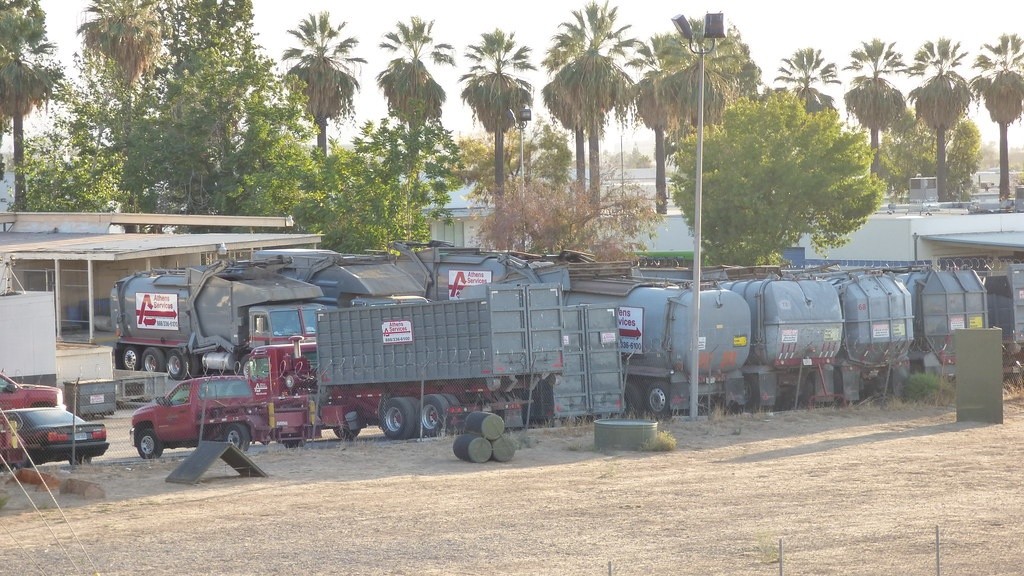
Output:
[247,281,565,442]
[107,245,1023,423]
[0,368,66,413]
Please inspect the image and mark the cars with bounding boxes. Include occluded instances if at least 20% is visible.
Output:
[0,407,110,471]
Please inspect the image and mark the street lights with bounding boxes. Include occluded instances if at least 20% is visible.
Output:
[515,107,532,244]
[669,9,731,423]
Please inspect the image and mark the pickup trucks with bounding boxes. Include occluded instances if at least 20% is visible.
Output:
[129,374,311,461]
